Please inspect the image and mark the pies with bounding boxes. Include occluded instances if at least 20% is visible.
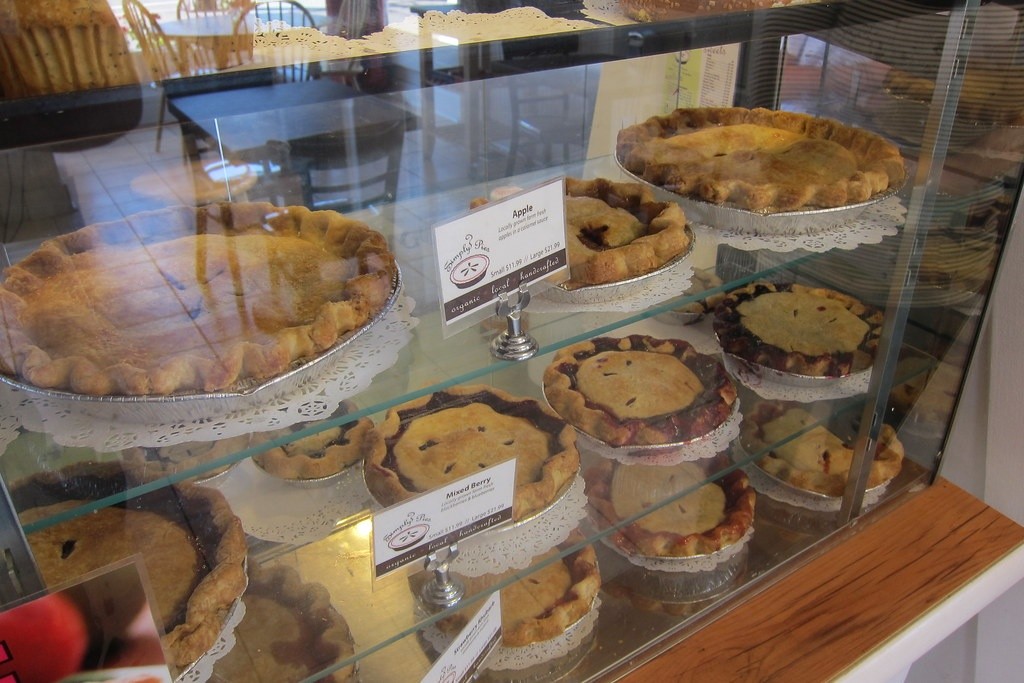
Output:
[1,1,1023,683]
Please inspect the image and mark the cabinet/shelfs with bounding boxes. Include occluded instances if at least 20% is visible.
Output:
[0,1,1024,681]
